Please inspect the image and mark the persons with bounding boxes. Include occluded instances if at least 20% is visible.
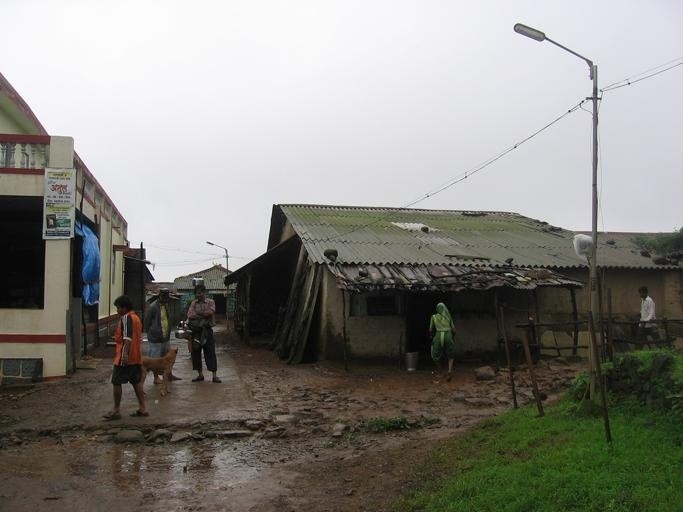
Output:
[184,285,222,384]
[101,294,148,420]
[633,285,661,351]
[427,301,456,384]
[142,287,182,384]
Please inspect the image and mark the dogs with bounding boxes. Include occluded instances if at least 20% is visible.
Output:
[141,346,178,396]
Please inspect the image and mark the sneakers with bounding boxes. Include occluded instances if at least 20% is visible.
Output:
[190,376,206,382]
[212,377,222,384]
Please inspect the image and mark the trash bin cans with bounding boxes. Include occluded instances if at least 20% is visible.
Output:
[406,352,419,372]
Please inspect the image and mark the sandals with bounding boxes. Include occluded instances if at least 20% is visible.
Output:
[102,411,121,419]
[128,410,150,417]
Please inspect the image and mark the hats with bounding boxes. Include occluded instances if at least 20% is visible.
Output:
[158,288,171,294]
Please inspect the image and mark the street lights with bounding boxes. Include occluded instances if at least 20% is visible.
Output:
[207,241,229,331]
[514,22,602,400]
